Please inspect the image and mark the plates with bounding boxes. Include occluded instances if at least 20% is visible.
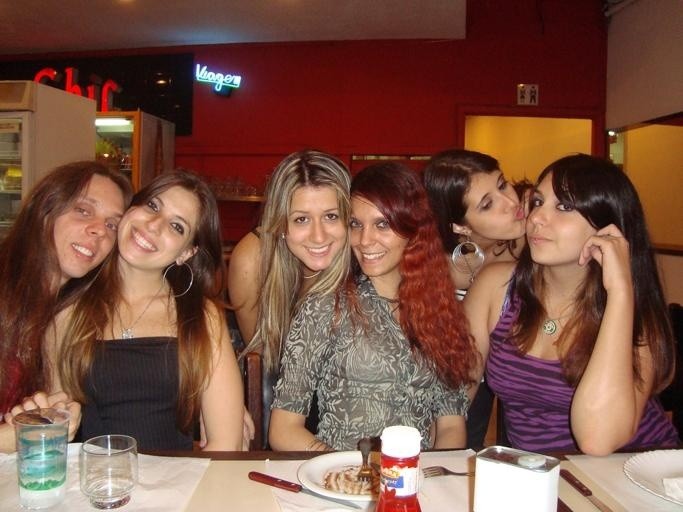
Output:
[623,448,683,506]
[296,450,381,502]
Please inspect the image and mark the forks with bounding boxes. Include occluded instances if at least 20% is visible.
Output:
[357,437,373,482]
[422,466,475,478]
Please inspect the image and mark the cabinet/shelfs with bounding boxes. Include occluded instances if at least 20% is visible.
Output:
[0,80,96,245]
[94,111,174,201]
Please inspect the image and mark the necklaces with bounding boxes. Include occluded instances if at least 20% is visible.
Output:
[542,313,571,335]
[462,254,475,284]
[105,281,169,339]
[302,268,322,279]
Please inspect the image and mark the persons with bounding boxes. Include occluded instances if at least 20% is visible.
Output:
[429,154,681,456]
[269,161,484,451]
[228,149,368,451]
[417,149,535,448]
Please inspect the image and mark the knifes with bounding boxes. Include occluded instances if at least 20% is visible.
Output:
[248,472,363,508]
[559,467,613,512]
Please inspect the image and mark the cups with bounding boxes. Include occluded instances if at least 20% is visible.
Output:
[80,434,138,510]
[12,408,71,510]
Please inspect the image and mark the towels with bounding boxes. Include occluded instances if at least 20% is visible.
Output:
[266,449,478,512]
[0,442,211,512]
[565,451,683,512]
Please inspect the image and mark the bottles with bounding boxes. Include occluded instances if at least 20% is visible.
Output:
[376,426,422,511]
